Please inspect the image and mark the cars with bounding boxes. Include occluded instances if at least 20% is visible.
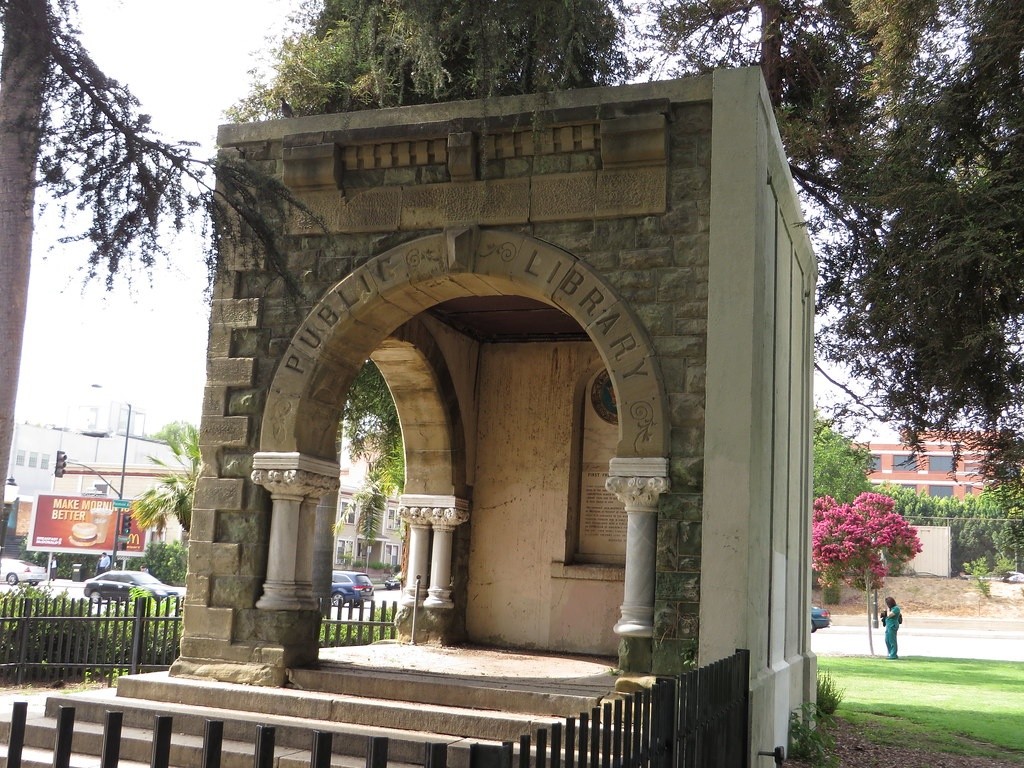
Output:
[811,606,831,634]
[0,557,47,586]
[384,570,403,590]
[83,569,185,606]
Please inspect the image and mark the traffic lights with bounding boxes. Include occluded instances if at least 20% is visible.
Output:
[54,450,67,479]
[121,512,132,537]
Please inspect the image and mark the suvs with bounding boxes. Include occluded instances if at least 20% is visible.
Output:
[332,570,375,607]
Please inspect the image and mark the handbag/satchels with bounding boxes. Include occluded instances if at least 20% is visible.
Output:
[884,611,902,624]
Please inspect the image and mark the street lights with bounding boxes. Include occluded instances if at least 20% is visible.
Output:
[91,384,131,570]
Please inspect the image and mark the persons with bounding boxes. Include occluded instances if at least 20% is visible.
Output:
[51,555,58,581]
[97,552,110,576]
[880,597,901,660]
[140,566,150,574]
